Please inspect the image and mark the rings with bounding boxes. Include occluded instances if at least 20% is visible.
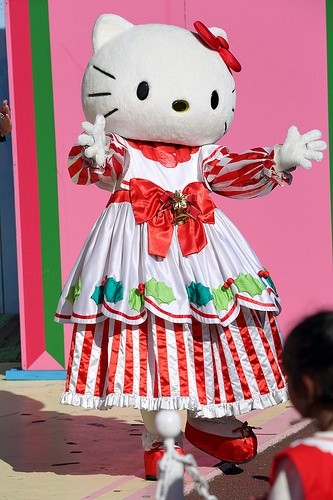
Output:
[305,143,309,149]
[91,134,96,144]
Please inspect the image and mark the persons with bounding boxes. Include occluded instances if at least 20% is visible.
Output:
[0,97,13,142]
[259,311,333,500]
[52,9,328,481]
